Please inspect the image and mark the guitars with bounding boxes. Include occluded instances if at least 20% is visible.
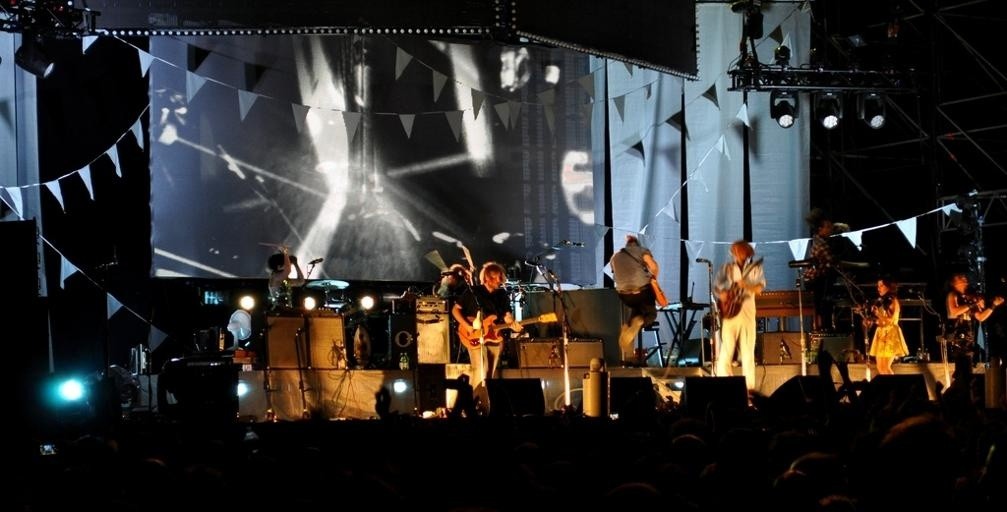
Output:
[458,312,558,349]
[717,258,763,319]
[650,275,668,307]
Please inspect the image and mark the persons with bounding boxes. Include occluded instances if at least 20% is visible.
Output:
[862,275,910,375]
[265,240,304,306]
[711,241,767,405]
[801,208,841,330]
[450,261,525,390]
[438,262,473,300]
[608,236,660,358]
[945,273,1005,373]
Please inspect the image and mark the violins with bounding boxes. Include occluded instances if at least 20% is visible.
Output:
[871,292,894,319]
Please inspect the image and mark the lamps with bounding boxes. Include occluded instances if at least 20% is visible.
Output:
[12,30,57,80]
[765,90,886,131]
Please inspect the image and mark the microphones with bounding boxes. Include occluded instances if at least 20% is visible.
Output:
[308,258,323,264]
[566,241,585,248]
[525,260,543,268]
[441,271,459,276]
[788,259,816,268]
[696,258,710,263]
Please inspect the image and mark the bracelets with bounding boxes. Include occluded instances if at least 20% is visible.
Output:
[989,302,995,309]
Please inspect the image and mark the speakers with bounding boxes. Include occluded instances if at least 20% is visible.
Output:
[857,374,930,406]
[768,375,838,412]
[809,330,855,364]
[610,377,658,413]
[265,311,309,370]
[415,364,445,416]
[763,331,807,365]
[474,378,546,417]
[680,375,748,415]
[388,313,451,370]
[308,311,347,370]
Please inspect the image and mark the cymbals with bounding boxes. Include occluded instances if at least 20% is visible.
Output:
[306,280,350,289]
